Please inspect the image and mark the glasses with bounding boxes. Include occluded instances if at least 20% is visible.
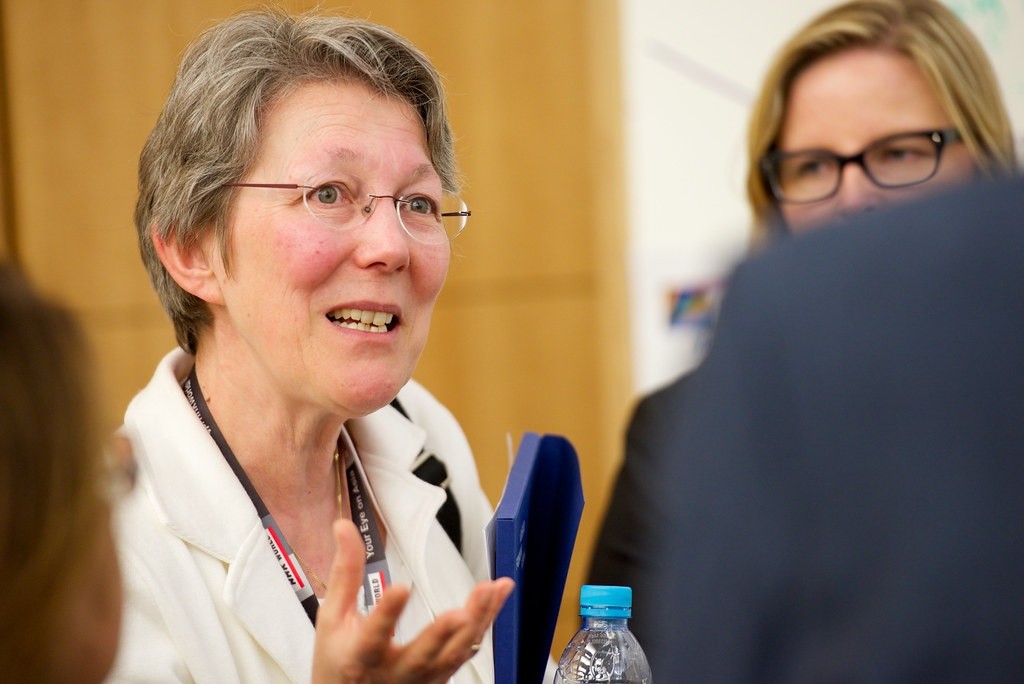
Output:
[92,435,138,500]
[210,172,473,246]
[757,128,965,204]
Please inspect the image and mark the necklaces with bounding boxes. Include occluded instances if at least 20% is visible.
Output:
[201,388,343,591]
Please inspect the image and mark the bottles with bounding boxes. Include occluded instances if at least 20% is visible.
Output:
[553,585,653,684]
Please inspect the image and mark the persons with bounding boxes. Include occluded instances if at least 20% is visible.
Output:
[0,261,137,684]
[575,0,1020,632]
[108,9,562,684]
[636,166,1024,684]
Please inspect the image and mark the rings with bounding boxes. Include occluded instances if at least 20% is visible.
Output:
[473,643,480,651]
